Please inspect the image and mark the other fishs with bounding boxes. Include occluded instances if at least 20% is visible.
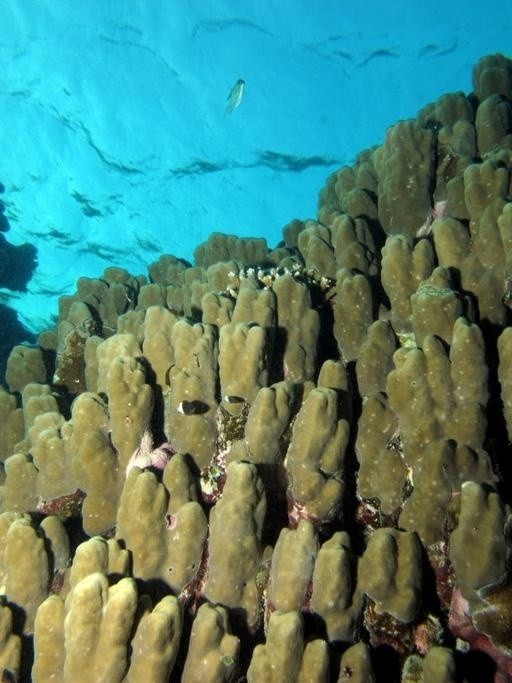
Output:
[225,78,246,113]
